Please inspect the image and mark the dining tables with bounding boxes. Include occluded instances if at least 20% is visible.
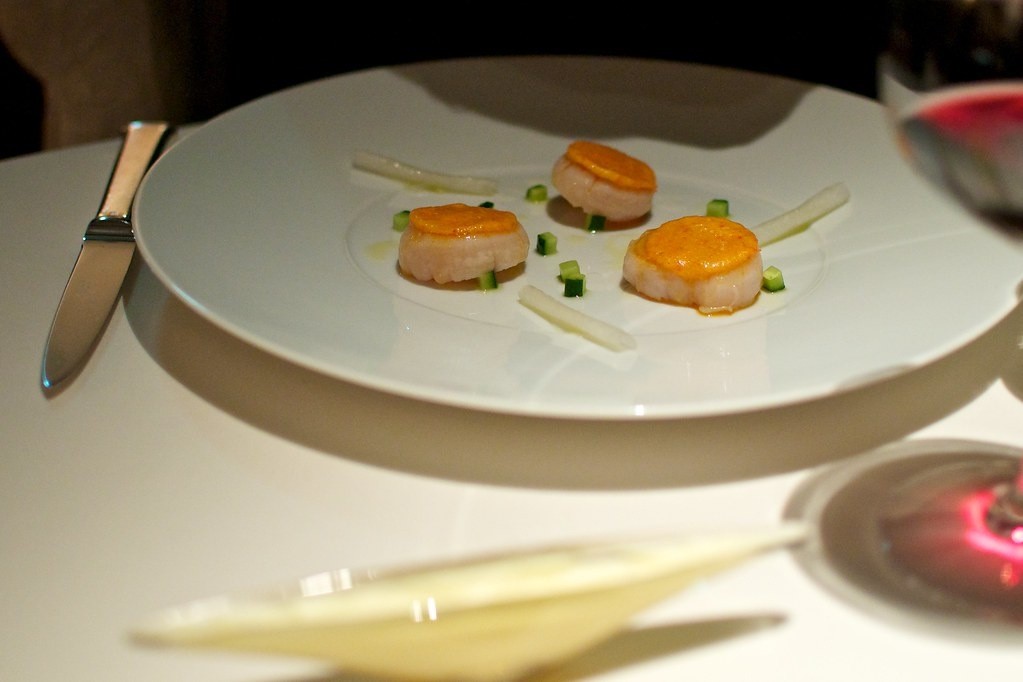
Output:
[0,124,1022,682]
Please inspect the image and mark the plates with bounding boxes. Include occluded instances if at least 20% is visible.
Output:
[131,55,1023,420]
[123,520,809,682]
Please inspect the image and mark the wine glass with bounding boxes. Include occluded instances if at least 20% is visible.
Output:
[875,0,1023,610]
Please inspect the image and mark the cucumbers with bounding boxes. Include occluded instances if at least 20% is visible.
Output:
[392,184,786,297]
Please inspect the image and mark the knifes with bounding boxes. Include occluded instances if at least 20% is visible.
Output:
[41,119,173,391]
